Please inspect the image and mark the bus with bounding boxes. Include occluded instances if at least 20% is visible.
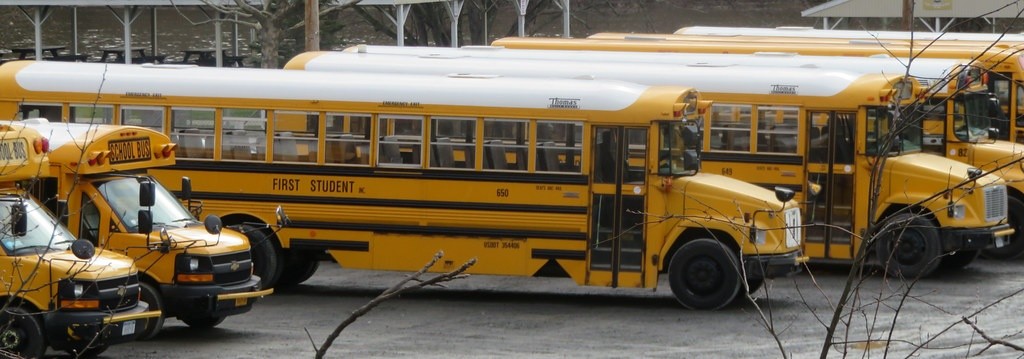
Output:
[0,60,803,312]
[0,119,275,359]
[284,26,1024,279]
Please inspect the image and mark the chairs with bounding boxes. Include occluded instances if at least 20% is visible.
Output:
[524,142,561,171]
[469,140,508,170]
[412,136,454,167]
[711,123,796,154]
[227,130,253,160]
[603,131,645,182]
[811,127,829,155]
[256,130,299,161]
[332,133,403,164]
[179,129,213,158]
[306,130,330,161]
